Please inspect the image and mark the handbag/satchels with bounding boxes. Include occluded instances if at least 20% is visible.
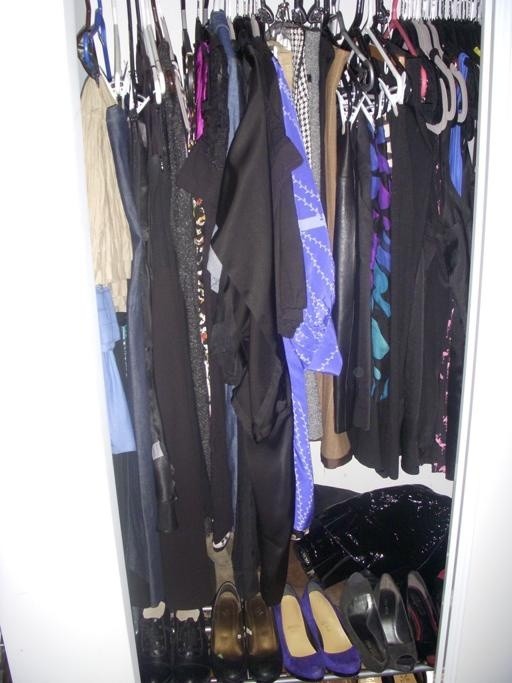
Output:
[293,483,452,590]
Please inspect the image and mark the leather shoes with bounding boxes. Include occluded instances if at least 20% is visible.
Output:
[373,572,418,672]
[244,591,283,682]
[338,571,390,672]
[272,582,326,681]
[404,569,440,667]
[210,580,248,681]
[299,580,362,675]
[175,607,211,682]
[138,600,173,682]
